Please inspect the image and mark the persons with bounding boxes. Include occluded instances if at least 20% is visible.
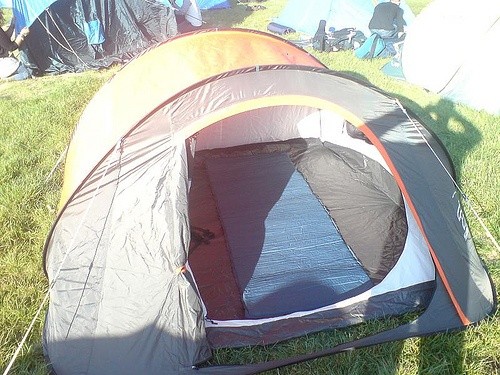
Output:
[0,1,33,82]
[169,0,206,34]
[372,0,407,59]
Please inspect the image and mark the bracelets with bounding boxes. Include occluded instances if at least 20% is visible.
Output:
[18,31,27,40]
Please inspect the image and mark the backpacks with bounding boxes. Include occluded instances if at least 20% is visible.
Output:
[312,27,385,61]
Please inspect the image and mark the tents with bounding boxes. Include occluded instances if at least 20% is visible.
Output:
[366,1,499,114]
[8,0,178,76]
[38,21,498,373]
[265,0,410,49]
